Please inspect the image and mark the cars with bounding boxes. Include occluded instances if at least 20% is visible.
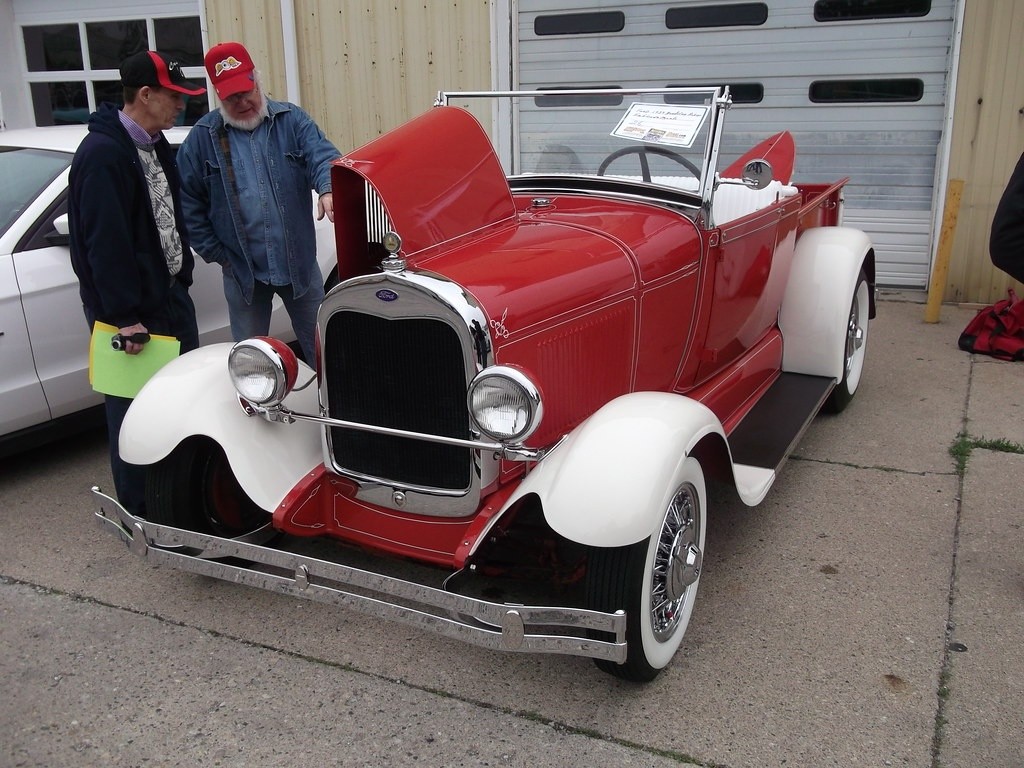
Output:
[0,123,336,465]
[92,86,877,681]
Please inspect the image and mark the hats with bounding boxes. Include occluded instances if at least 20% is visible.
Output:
[205,42,255,99]
[119,51,206,96]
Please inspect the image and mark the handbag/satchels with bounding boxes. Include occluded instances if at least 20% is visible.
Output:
[958,288,1024,361]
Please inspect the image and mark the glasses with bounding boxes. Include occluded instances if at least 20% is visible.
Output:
[226,80,256,103]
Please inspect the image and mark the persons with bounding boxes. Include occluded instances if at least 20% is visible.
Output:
[174,40,344,374]
[65,48,215,499]
